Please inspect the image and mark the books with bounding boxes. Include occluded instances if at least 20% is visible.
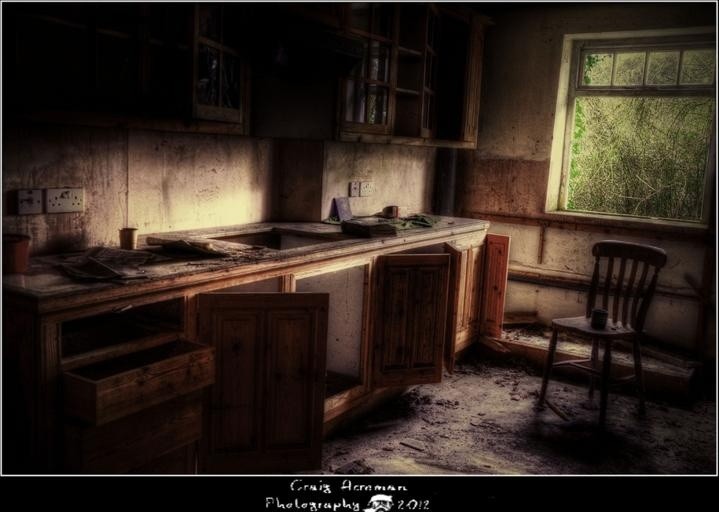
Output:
[341,217,398,239]
[332,196,353,224]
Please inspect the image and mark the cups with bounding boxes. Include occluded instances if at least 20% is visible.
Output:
[3,232,31,276]
[590,308,609,330]
[120,227,138,251]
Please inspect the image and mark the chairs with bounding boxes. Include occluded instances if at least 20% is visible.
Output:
[535,238,667,429]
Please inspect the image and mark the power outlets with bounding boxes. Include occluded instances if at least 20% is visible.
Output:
[359,181,375,197]
[45,186,87,214]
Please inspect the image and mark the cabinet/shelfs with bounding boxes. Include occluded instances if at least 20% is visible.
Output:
[365,230,511,407]
[199,257,375,478]
[2,293,217,476]
